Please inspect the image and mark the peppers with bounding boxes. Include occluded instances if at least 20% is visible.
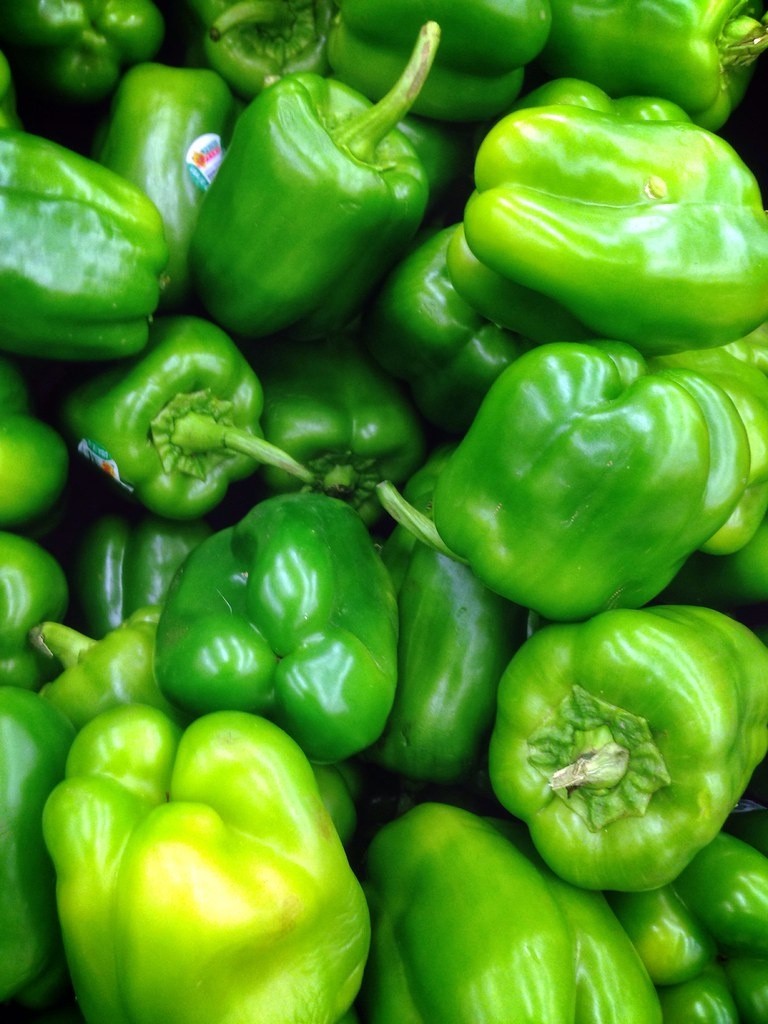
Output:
[0,0,768,1024]
